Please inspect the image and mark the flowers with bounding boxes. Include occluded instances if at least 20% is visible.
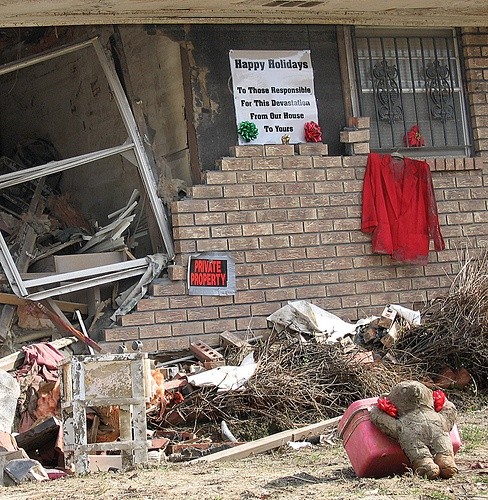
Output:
[303,121,323,142]
[238,121,258,142]
[402,125,424,147]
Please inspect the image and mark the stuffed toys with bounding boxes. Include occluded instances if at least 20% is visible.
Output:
[370,380,457,480]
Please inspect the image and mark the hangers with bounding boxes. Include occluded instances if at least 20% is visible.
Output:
[369,145,430,170]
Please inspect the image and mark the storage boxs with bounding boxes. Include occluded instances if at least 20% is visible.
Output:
[338,397,462,477]
